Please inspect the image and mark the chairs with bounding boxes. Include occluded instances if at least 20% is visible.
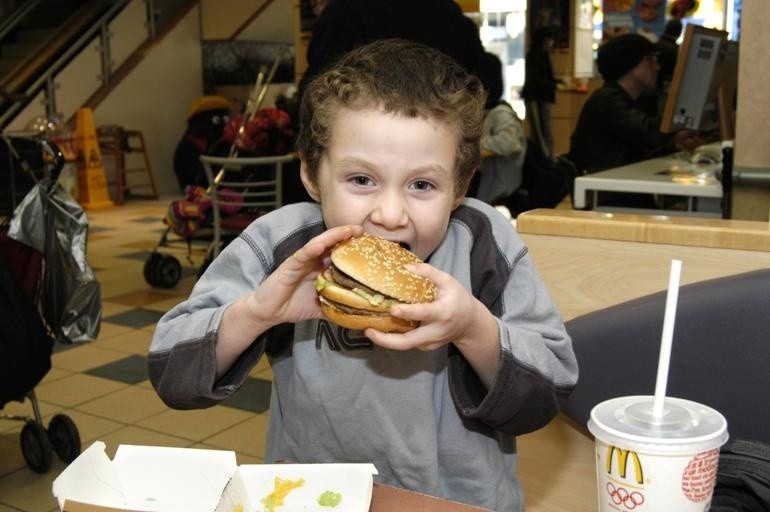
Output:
[195,152,296,266]
[96,122,160,206]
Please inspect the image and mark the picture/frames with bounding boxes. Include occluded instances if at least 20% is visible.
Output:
[659,23,729,133]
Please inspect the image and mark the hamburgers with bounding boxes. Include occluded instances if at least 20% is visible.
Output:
[316,232,438,333]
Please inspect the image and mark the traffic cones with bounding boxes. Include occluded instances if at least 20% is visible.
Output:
[71,108,116,212]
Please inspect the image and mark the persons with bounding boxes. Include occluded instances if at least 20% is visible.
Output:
[146,39,580,512]
[294,0,739,209]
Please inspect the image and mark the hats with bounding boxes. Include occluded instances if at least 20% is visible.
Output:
[597,33,656,82]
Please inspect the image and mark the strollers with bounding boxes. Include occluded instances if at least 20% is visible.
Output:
[142,42,316,289]
[0,130,103,474]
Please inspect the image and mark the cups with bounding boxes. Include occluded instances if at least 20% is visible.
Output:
[585,396,732,512]
